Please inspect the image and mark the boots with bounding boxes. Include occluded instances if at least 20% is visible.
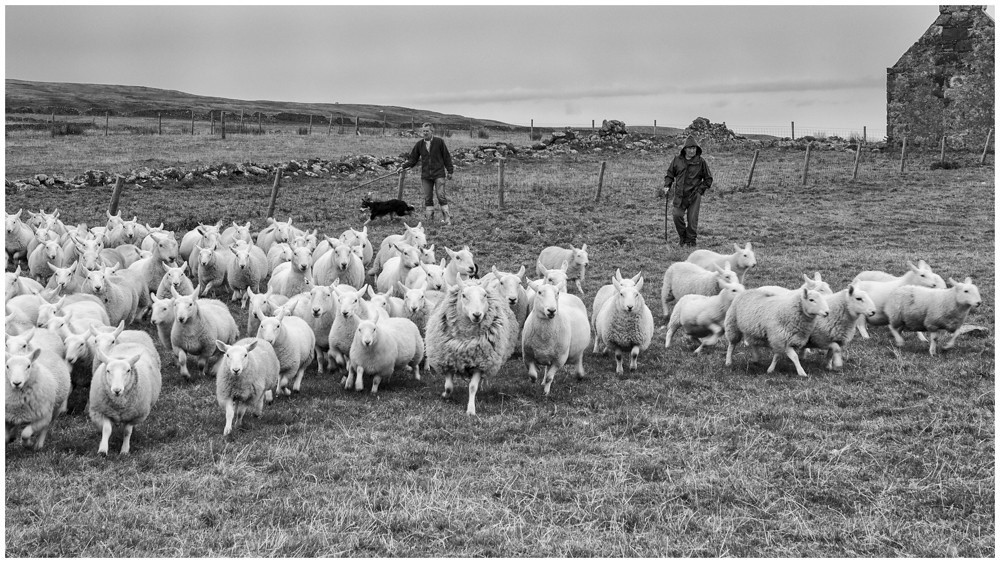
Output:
[441,204,451,225]
[424,205,434,221]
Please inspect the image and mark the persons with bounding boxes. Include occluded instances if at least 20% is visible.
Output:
[397,122,453,225]
[663,134,713,247]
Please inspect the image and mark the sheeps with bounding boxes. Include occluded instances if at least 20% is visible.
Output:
[87,342,162,456]
[6,205,644,449]
[662,279,745,354]
[424,270,519,417]
[215,338,280,436]
[882,275,980,357]
[534,244,591,295]
[724,289,829,377]
[757,259,950,372]
[169,285,236,380]
[660,258,742,317]
[348,311,398,396]
[595,276,656,374]
[685,242,757,274]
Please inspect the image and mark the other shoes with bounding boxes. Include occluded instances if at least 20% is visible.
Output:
[682,239,696,246]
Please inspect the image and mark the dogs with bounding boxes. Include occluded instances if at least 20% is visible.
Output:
[360,197,414,225]
